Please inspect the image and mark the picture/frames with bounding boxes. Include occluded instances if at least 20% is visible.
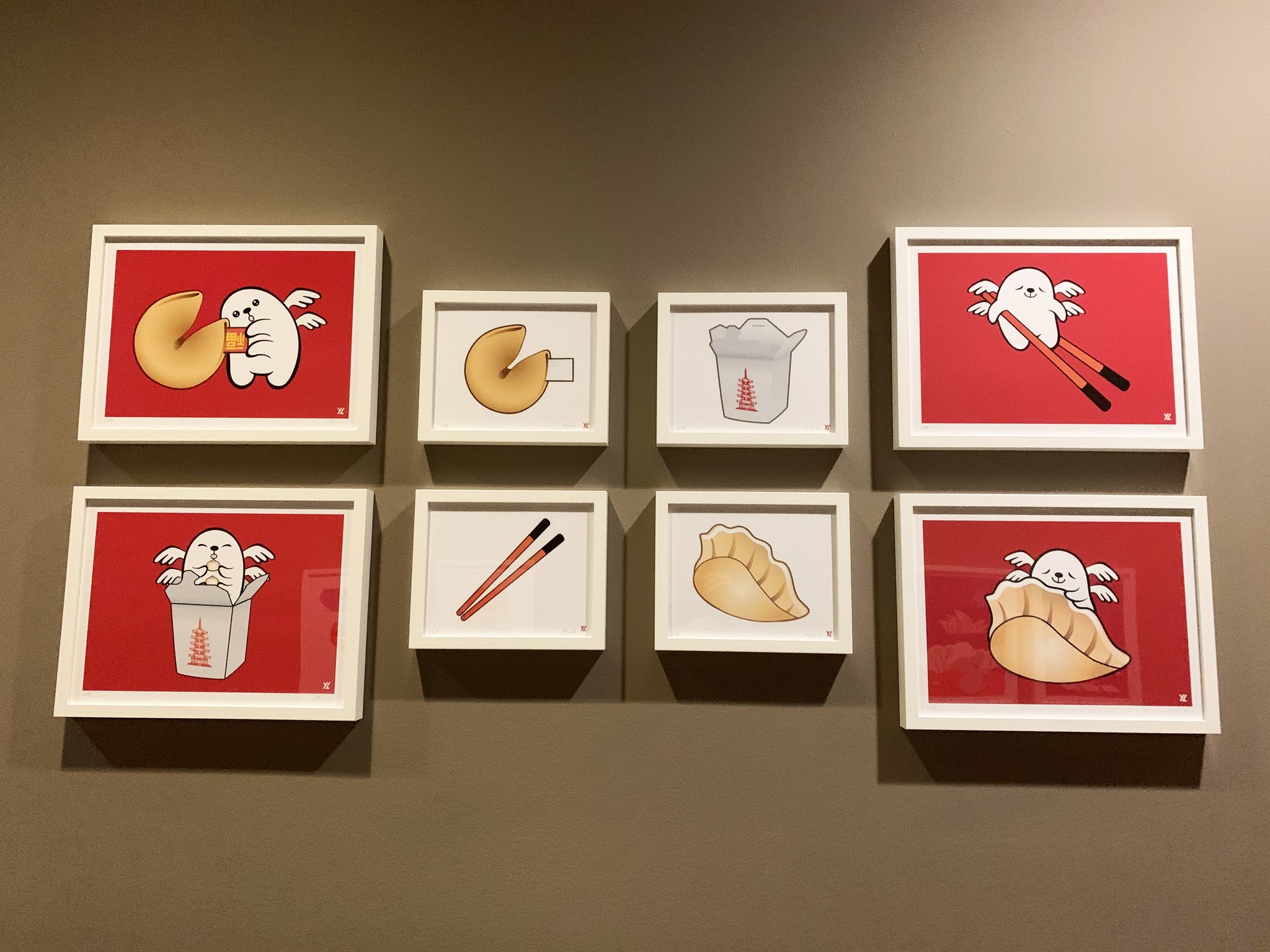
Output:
[652,487,856,657]
[413,284,612,450]
[74,217,390,447]
[887,223,1209,458]
[893,485,1225,742]
[654,287,854,449]
[51,478,379,724]
[405,481,609,653]
